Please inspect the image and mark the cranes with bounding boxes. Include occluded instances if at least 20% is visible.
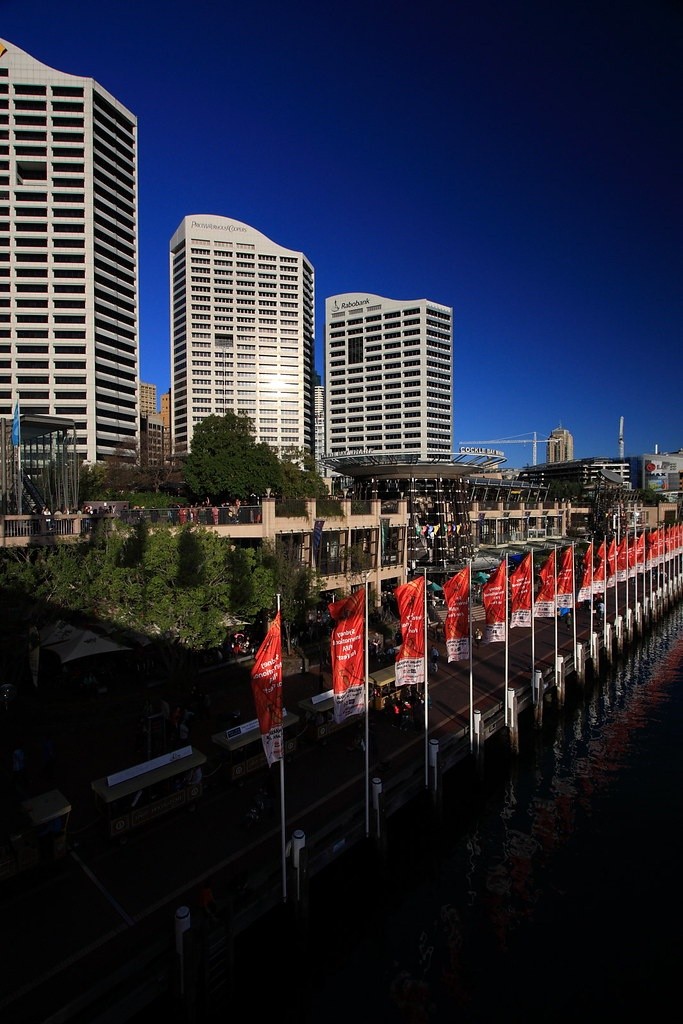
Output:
[458,432,564,466]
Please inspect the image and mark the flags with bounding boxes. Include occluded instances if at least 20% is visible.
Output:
[481,546,575,645]
[576,522,683,600]
[393,577,430,687]
[250,611,283,766]
[327,588,367,723]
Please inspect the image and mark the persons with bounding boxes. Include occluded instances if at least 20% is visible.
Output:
[556,567,667,637]
[10,732,63,785]
[26,489,343,535]
[307,592,484,751]
[197,878,251,928]
[76,635,295,692]
[131,692,333,827]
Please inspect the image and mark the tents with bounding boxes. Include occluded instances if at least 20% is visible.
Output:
[35,606,251,692]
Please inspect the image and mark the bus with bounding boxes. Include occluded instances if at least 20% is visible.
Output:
[0,664,421,890]
